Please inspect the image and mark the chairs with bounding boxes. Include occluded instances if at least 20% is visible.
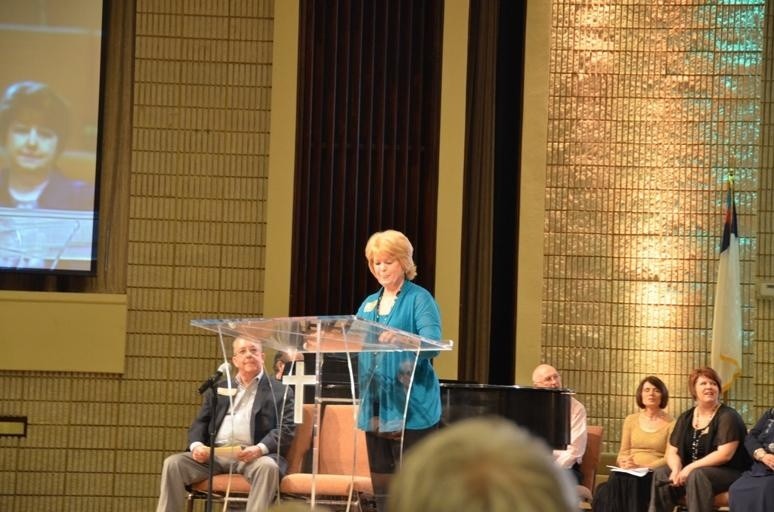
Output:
[575,425,605,511]
[187,404,374,512]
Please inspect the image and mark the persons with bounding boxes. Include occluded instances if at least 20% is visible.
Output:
[591,375,678,511]
[0,81,94,211]
[303,229,443,512]
[269,347,305,381]
[532,363,588,486]
[383,415,581,512]
[647,366,750,512]
[728,404,774,511]
[155,332,296,512]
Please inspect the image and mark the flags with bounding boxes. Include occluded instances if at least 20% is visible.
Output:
[710,180,743,395]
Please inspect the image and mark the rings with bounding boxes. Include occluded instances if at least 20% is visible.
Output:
[244,459,249,463]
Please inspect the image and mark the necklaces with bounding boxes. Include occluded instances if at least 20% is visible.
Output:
[691,404,718,463]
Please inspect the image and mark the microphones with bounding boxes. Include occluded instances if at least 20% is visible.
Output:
[198,362,232,394]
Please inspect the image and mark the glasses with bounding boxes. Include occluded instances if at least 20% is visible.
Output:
[536,374,561,383]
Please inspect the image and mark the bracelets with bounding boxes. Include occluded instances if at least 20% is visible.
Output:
[753,448,766,460]
